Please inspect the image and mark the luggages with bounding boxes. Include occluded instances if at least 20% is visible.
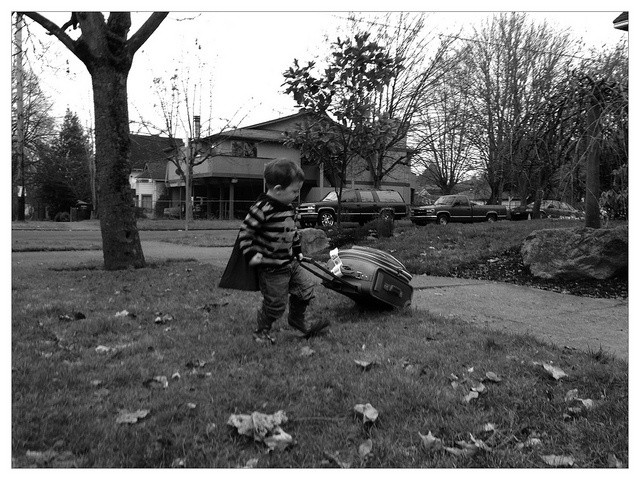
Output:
[299,245,413,309]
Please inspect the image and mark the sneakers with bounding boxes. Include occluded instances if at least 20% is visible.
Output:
[289,318,311,333]
[254,332,277,344]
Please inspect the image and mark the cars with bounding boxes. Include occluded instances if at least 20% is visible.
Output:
[512,200,582,220]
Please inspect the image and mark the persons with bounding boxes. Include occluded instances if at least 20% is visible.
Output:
[239,158,315,343]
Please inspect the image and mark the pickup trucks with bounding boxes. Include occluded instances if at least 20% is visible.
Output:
[409,195,509,226]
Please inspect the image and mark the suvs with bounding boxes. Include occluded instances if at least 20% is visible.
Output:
[296,189,409,228]
